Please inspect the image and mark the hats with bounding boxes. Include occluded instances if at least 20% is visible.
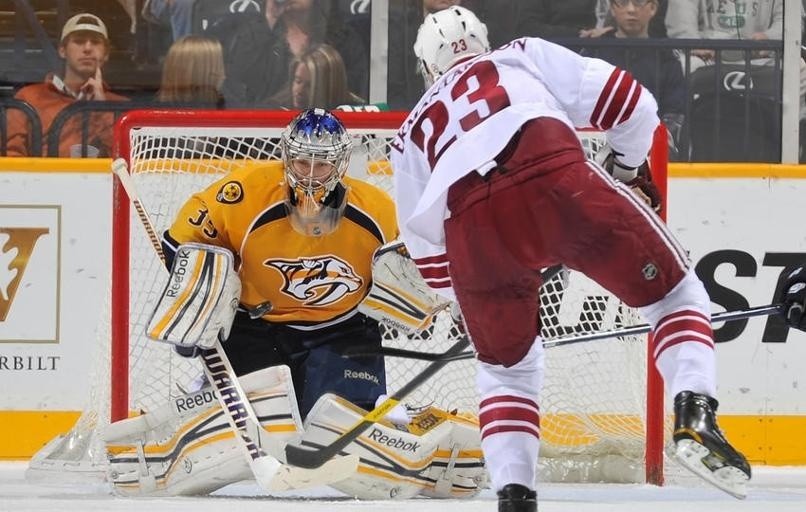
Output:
[60,13,108,44]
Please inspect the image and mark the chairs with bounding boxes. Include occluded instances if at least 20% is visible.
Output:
[684,58,782,166]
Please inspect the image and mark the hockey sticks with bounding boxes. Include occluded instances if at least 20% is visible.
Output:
[111,158,359,490]
[341,304,783,360]
[245,263,564,469]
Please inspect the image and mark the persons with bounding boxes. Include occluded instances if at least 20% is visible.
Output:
[160,113,436,464]
[391,6,755,512]
[391,0,485,109]
[3,1,805,163]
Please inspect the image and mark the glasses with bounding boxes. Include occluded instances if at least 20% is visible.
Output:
[613,0,650,7]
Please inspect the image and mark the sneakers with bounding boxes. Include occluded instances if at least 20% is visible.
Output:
[496,483,538,512]
[673,390,752,481]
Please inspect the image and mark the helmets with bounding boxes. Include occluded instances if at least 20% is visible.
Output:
[413,4,490,92]
[280,107,354,207]
